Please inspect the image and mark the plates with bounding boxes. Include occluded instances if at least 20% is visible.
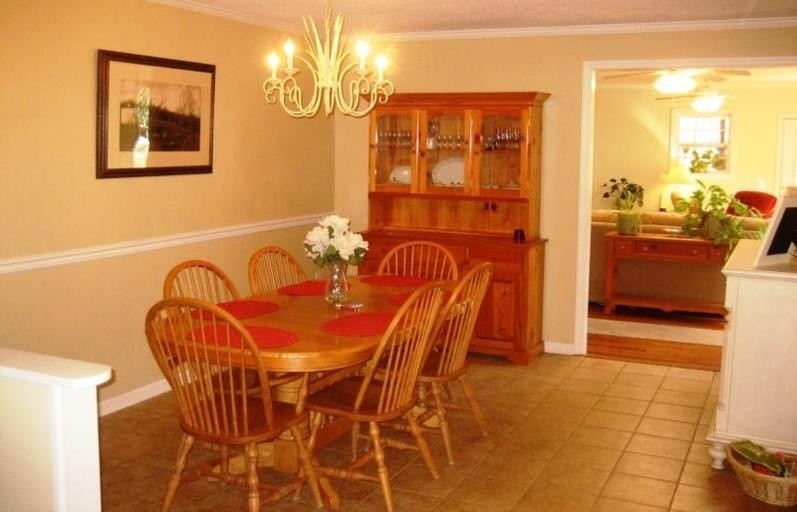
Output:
[429,155,467,190]
[387,163,412,185]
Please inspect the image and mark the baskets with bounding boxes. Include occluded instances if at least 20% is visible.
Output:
[723,441,797,509]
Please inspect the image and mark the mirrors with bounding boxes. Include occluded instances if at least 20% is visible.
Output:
[666,108,735,186]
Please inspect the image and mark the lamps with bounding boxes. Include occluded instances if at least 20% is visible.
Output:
[257,0,394,121]
[651,74,698,96]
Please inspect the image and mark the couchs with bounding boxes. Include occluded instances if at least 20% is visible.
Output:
[587,208,770,307]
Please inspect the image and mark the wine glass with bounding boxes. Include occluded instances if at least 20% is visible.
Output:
[378,127,412,150]
[427,119,464,152]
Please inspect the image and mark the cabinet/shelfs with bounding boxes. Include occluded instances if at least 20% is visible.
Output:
[702,236,797,472]
[353,82,551,246]
[350,229,549,368]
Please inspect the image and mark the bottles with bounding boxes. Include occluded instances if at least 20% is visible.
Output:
[481,124,523,153]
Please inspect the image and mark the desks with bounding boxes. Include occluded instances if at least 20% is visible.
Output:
[601,229,735,324]
[150,274,482,510]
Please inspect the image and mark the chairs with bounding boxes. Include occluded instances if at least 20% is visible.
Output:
[289,282,445,510]
[724,189,776,217]
[374,237,461,283]
[369,259,498,464]
[158,258,273,395]
[247,242,308,292]
[140,295,327,511]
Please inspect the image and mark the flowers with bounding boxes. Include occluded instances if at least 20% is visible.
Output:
[301,211,370,267]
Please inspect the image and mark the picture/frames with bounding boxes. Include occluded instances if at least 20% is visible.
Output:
[93,46,217,180]
[749,184,796,268]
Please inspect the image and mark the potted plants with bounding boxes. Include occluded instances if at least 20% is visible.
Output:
[596,176,646,235]
[675,177,771,263]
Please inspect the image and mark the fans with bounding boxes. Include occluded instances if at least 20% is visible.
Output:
[603,70,751,81]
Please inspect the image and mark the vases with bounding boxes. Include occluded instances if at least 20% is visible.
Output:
[320,257,350,306]
